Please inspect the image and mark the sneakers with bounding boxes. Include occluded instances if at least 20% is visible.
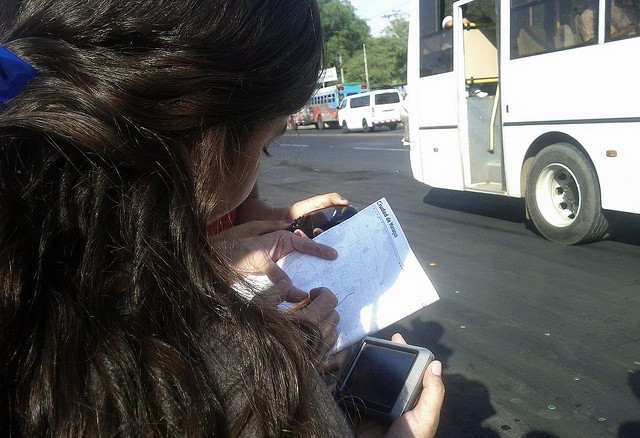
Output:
[470,88,488,98]
[466,85,470,97]
[403,141,410,146]
[401,138,405,143]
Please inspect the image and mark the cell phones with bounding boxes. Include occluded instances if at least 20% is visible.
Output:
[287,204,359,232]
[338,336,436,420]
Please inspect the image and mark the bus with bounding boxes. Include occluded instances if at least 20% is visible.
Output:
[288,82,370,130]
[408,1,640,245]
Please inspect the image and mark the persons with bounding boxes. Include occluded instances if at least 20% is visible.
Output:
[0,1,447,438]
[439,1,494,30]
[579,0,637,45]
[207,179,350,244]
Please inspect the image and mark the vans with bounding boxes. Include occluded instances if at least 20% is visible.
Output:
[336,88,402,132]
